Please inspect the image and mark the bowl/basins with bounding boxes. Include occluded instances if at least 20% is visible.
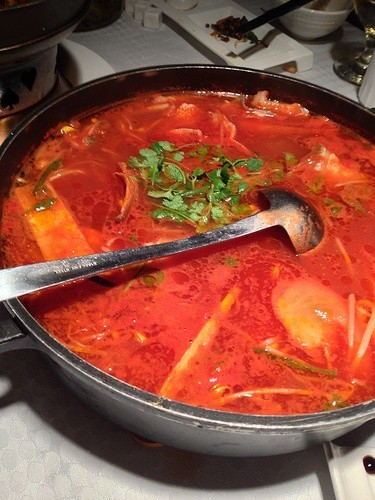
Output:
[274,0,353,41]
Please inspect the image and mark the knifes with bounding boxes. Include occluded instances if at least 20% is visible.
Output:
[237,0,312,36]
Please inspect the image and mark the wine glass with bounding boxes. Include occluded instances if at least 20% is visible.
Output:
[333,0,375,85]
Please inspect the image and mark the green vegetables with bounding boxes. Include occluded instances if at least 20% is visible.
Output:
[121,139,267,234]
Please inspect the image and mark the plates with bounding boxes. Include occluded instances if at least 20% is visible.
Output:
[0,39,115,146]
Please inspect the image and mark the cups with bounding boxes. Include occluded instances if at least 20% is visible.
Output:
[357,52,375,108]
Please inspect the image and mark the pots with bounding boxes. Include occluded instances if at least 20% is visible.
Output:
[0,65,374,458]
[0,0,93,119]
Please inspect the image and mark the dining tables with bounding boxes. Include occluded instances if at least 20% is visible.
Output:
[0,0,375,500]
